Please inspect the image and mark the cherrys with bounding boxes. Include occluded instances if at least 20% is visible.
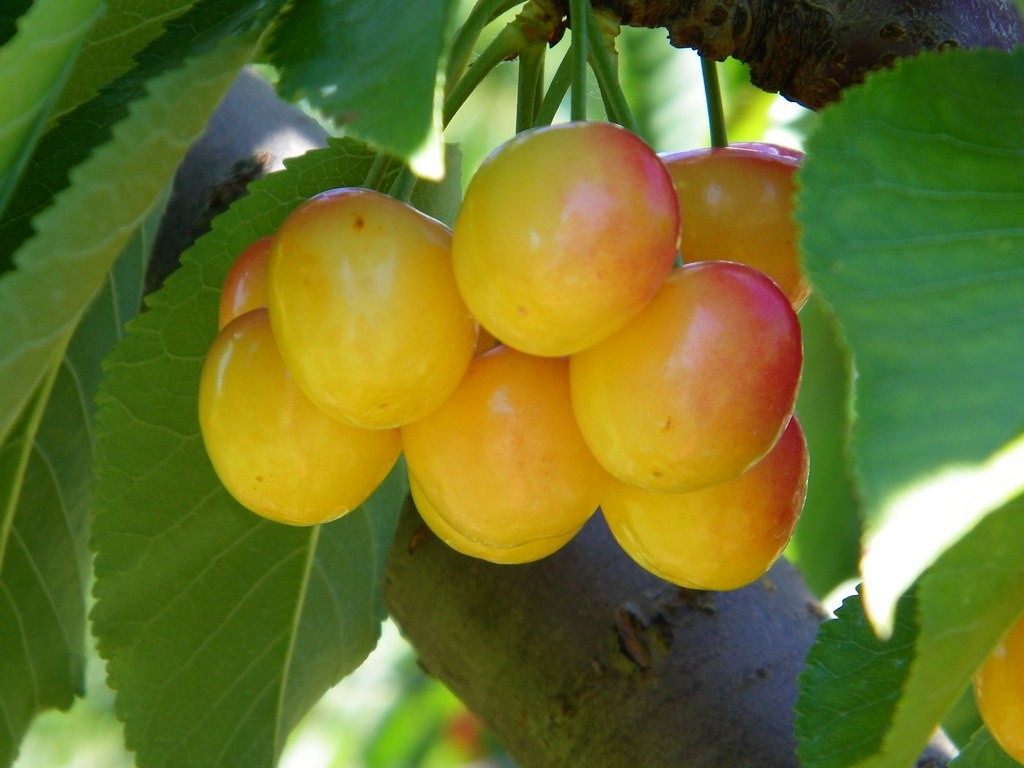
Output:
[971,608,1024,768]
[195,0,814,591]
